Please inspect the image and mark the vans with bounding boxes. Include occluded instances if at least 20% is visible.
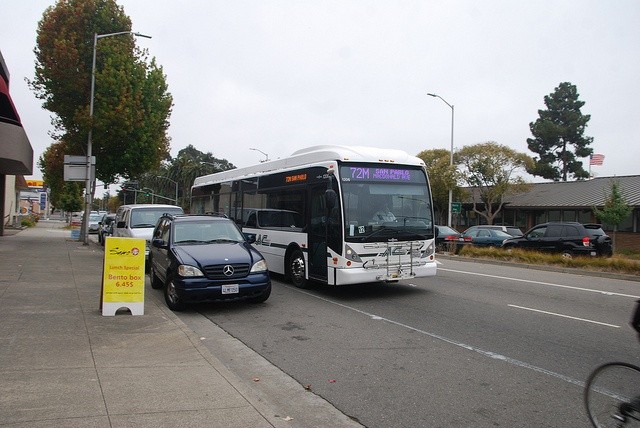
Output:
[112,204,184,269]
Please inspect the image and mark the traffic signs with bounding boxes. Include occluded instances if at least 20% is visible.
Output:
[451,202,461,214]
[427,93,454,228]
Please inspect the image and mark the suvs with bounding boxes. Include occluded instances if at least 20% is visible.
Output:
[148,213,271,310]
[501,221,612,262]
[461,226,522,249]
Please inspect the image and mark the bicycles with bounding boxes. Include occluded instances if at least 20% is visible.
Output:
[582,298,639,428]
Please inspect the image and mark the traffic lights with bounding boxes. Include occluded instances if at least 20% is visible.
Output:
[83,30,152,245]
[145,192,150,197]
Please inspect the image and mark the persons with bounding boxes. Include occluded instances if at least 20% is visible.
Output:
[374,204,396,222]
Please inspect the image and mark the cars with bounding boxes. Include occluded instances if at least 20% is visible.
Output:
[88,214,100,233]
[99,213,114,245]
[435,226,473,256]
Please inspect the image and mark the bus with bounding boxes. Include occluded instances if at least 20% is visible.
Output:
[189,144,436,289]
